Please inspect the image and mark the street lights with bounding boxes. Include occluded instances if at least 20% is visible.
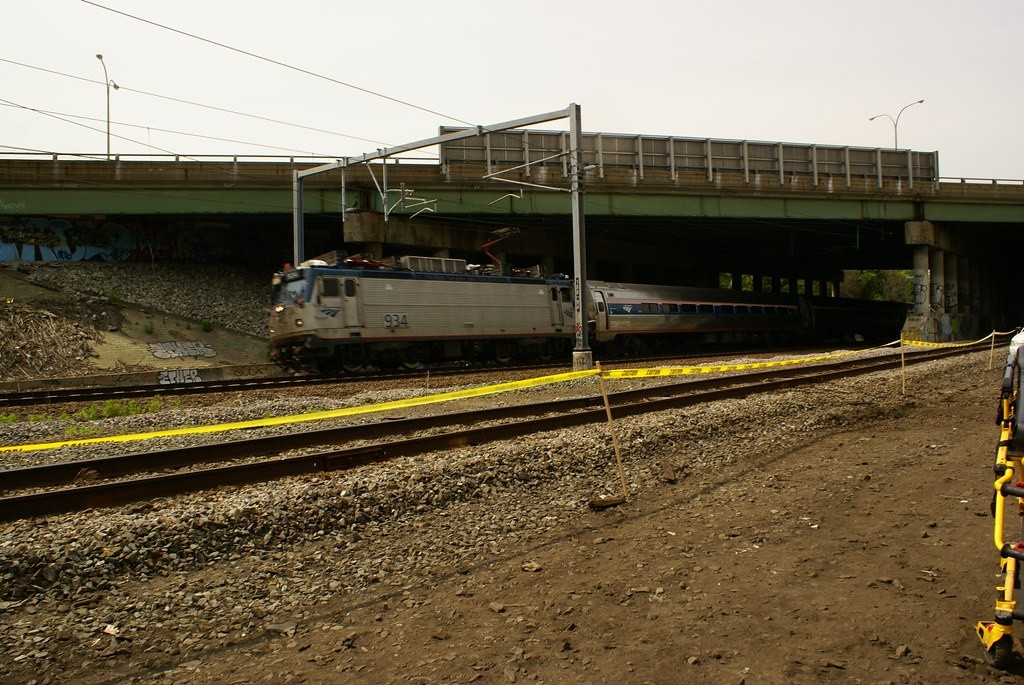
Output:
[96,54,119,160]
[869,97,927,150]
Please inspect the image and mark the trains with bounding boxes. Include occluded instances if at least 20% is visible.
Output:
[268,260,913,376]
